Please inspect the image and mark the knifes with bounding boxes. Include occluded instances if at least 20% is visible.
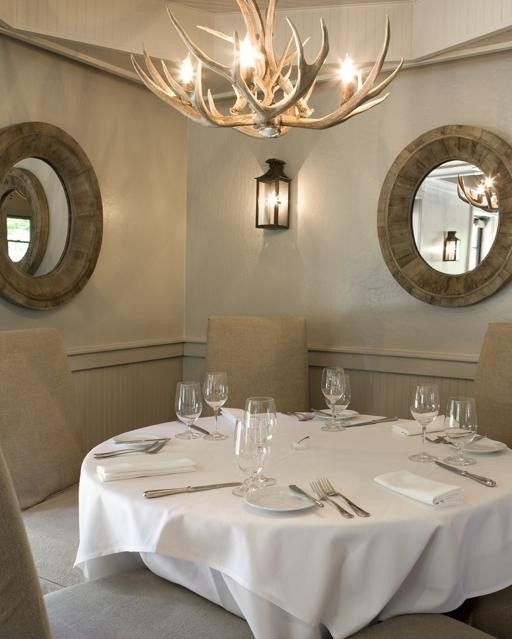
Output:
[177,419,209,435]
[435,461,496,487]
[342,415,398,428]
[290,484,324,508]
[143,482,243,499]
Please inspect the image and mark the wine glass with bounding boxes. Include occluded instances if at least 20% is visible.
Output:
[174,382,203,440]
[243,396,277,487]
[443,396,478,466]
[408,384,441,462]
[321,366,346,427]
[326,374,351,431]
[234,416,267,498]
[203,372,229,441]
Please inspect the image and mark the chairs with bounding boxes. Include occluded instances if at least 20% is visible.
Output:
[475,324,512,443]
[0,453,256,638]
[203,316,310,414]
[0,327,88,589]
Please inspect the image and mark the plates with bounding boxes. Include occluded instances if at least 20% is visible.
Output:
[448,438,507,454]
[243,485,318,512]
[313,408,359,420]
[114,434,160,445]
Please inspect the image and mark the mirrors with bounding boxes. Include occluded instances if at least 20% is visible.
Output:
[377,125,511,308]
[2,167,48,277]
[0,121,103,311]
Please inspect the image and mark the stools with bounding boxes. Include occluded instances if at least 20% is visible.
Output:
[468,591,512,639]
[345,613,502,639]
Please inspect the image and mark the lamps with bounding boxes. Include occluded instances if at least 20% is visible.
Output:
[131,0,404,137]
[253,158,292,229]
[443,231,460,261]
[455,173,499,213]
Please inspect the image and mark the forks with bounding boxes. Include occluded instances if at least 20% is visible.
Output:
[93,441,167,459]
[310,478,371,518]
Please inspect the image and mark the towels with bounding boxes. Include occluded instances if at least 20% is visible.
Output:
[220,406,246,425]
[391,415,458,436]
[374,472,466,507]
[96,454,198,483]
